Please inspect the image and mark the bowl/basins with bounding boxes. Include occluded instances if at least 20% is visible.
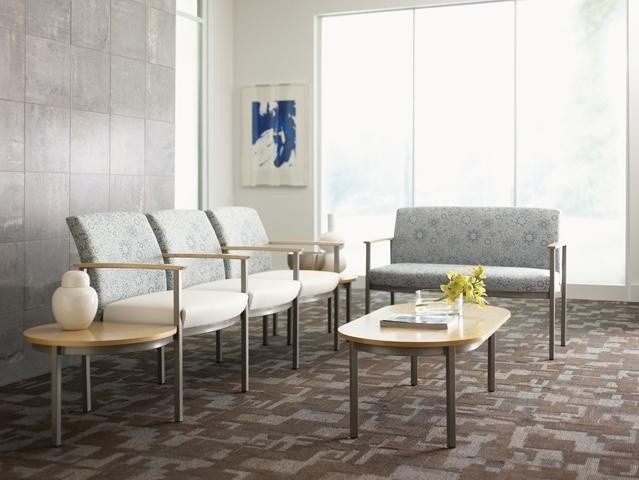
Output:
[287,252,325,270]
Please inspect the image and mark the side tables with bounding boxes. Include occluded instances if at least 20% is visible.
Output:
[22,321,177,446]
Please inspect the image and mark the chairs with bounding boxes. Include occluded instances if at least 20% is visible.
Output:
[147,207,303,369]
[206,206,354,352]
[67,212,250,422]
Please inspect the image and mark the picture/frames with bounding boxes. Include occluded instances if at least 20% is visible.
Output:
[238,83,309,188]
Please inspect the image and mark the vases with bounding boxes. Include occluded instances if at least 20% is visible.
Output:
[414,288,464,318]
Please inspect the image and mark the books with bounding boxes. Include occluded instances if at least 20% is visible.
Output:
[380,312,459,331]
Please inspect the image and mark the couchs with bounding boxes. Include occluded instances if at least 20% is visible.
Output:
[364,207,566,359]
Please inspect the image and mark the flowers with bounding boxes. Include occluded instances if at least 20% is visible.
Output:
[440,265,491,306]
